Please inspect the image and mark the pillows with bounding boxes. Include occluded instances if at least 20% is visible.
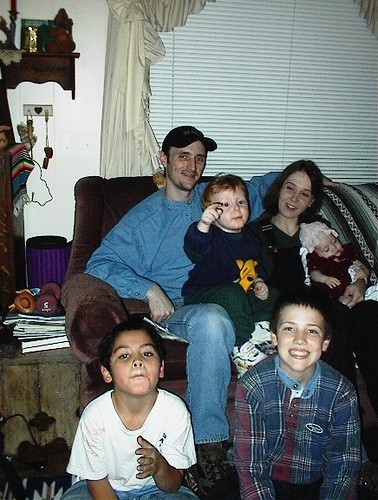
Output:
[317,183,378,286]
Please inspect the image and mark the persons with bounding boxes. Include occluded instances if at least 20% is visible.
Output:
[247,161,378,492]
[202,288,378,500]
[60,320,200,500]
[84,126,334,493]
[181,173,280,369]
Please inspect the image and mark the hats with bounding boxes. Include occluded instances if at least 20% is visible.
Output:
[299,221,339,254]
[164,125,217,152]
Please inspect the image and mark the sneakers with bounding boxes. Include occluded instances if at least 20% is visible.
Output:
[184,442,240,500]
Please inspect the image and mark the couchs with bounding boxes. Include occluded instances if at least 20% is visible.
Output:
[60,175,378,443]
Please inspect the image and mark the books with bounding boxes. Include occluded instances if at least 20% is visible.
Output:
[2,311,70,354]
